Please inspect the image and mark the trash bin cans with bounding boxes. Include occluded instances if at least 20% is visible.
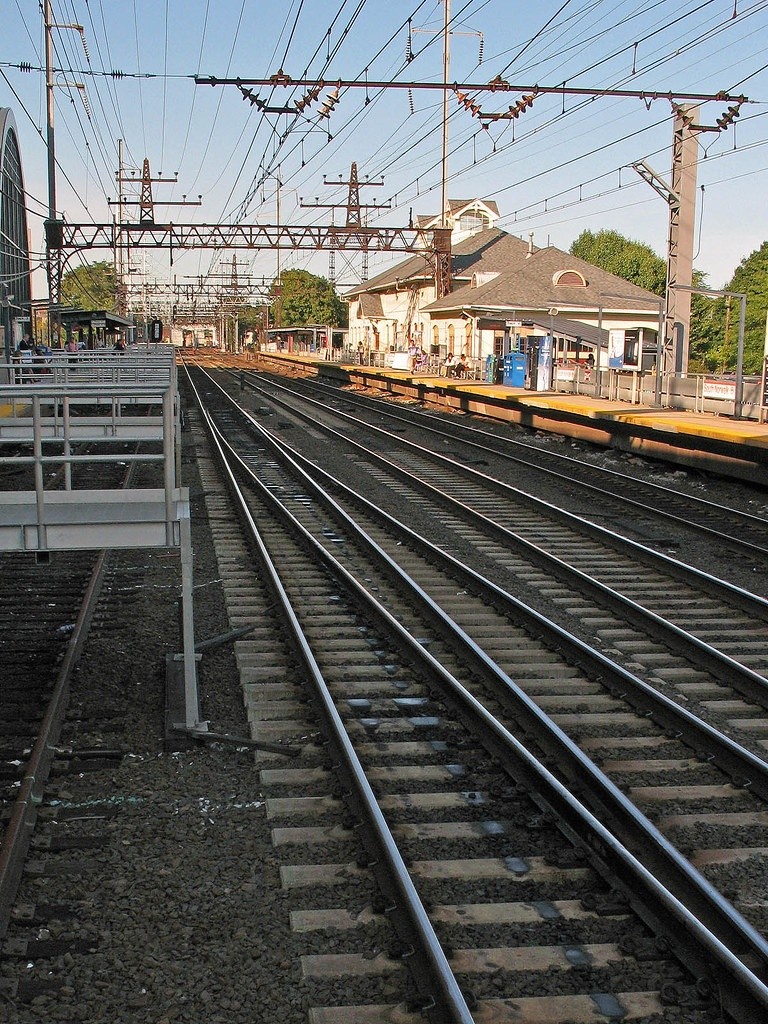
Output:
[503,353,526,388]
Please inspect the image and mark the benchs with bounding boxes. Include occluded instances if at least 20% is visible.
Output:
[417,359,476,381]
[334,351,381,367]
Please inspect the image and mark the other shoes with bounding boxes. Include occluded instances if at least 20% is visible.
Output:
[454,376,459,380]
[410,371,413,374]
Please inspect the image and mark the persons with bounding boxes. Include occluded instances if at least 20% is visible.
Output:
[587,354,595,369]
[65,336,78,371]
[409,340,417,374]
[443,353,459,378]
[13,350,41,384]
[415,350,428,371]
[20,333,54,374]
[455,354,471,379]
[348,343,352,351]
[112,339,126,356]
[357,342,364,366]
